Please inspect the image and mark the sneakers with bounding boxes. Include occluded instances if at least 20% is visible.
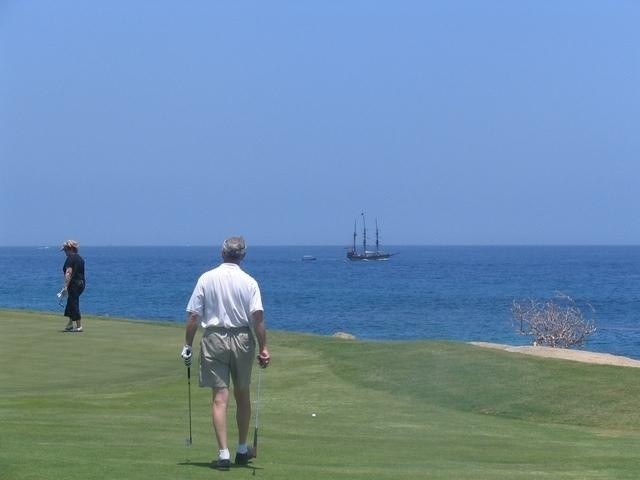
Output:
[235,447,256,465]
[217,459,230,467]
[64,326,82,332]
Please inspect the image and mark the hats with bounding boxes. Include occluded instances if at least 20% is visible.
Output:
[60,240,79,251]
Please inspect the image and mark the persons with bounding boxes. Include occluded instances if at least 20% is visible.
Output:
[60,240,85,332]
[181,237,270,469]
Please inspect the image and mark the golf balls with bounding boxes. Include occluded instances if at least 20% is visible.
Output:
[312,413,316,417]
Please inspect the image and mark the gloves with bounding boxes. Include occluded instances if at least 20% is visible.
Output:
[182,344,192,361]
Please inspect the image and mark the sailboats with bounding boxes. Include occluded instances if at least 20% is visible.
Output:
[344,212,400,262]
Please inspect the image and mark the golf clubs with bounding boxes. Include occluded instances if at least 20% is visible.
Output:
[247,359,261,457]
[58,272,72,308]
[186,350,192,447]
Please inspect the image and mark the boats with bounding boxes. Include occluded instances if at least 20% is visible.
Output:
[302,255,316,261]
